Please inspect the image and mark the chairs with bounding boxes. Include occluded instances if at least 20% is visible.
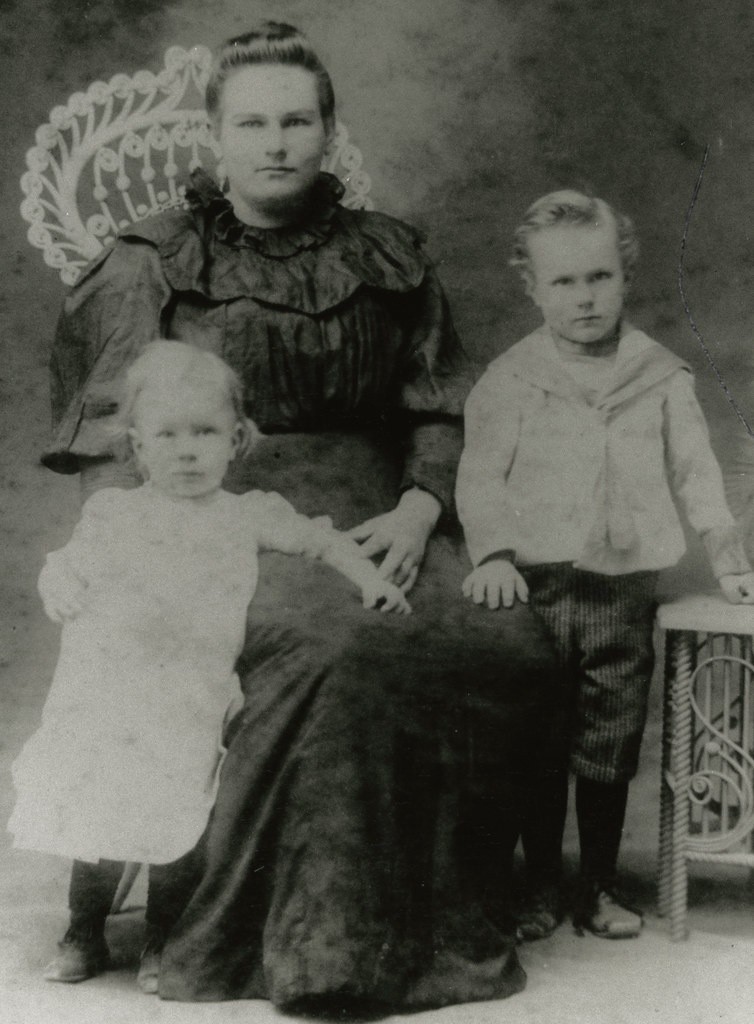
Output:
[23,45,373,289]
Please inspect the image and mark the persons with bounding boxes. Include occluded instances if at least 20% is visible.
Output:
[37,17,529,1013]
[43,336,414,995]
[453,191,754,939]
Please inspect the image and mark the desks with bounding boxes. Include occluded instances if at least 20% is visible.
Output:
[657,590,754,943]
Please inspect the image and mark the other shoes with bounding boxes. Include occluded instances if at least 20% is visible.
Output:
[41,939,96,981]
[136,944,161,992]
[511,890,569,938]
[585,888,644,938]
